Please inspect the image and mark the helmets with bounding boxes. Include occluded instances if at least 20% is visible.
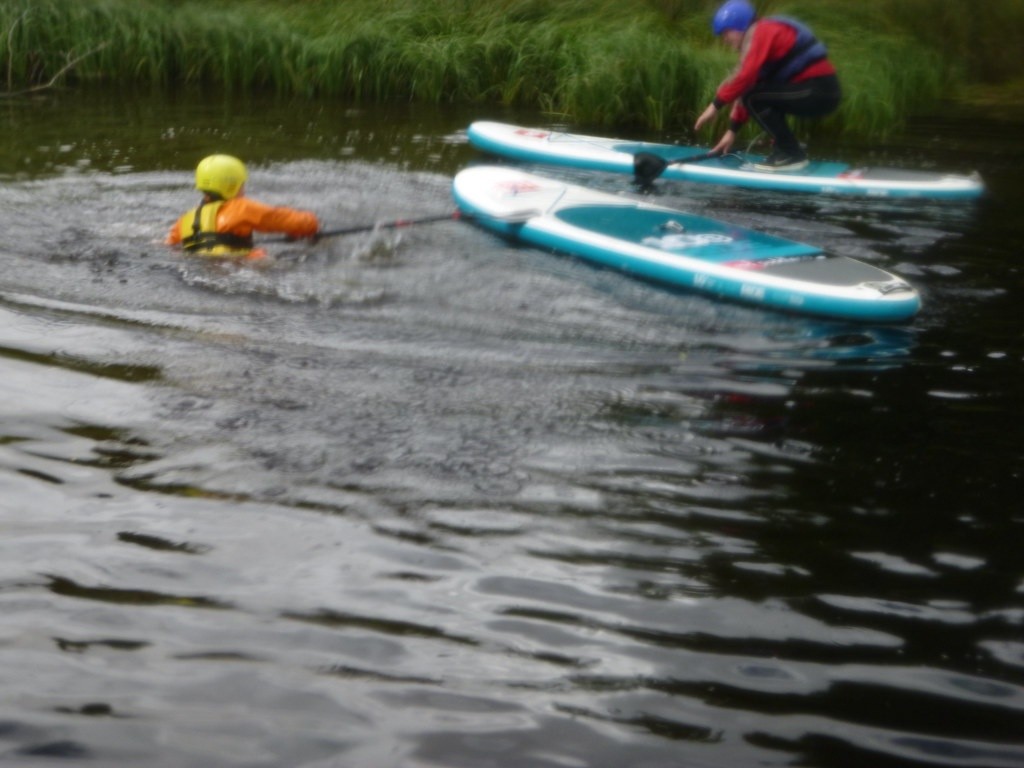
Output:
[713,0,755,35]
[194,154,246,200]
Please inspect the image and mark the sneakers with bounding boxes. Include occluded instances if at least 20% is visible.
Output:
[755,152,810,172]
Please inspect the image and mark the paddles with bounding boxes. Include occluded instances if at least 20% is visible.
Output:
[258,210,467,244]
[632,144,721,186]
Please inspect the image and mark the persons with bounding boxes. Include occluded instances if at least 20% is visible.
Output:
[694,0,842,171]
[167,155,318,261]
